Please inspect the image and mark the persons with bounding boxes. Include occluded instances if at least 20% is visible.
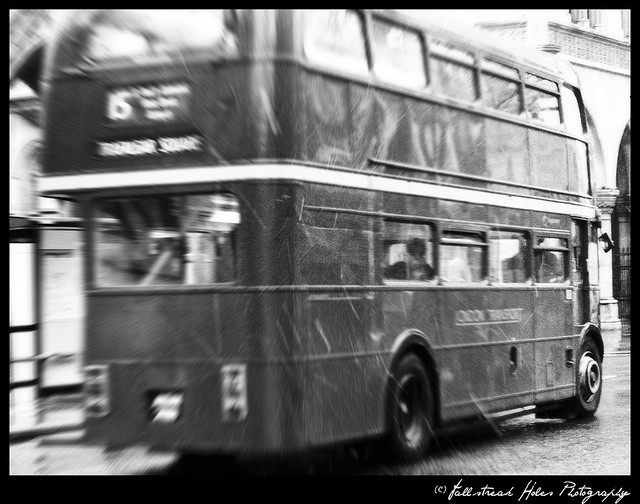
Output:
[382,237,435,281]
[507,230,558,283]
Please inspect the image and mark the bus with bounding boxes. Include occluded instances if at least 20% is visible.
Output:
[33,9,615,462]
